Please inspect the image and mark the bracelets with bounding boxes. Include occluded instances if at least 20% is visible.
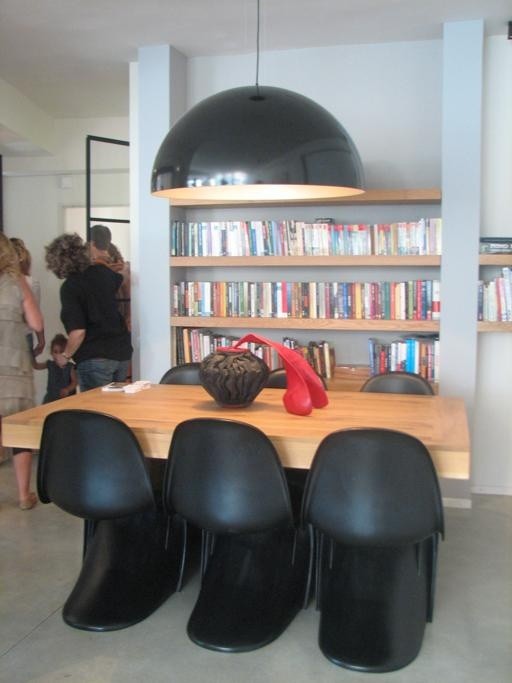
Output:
[126,375,132,379]
[62,352,70,360]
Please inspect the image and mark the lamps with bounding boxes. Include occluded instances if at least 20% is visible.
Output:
[151,0,366,202]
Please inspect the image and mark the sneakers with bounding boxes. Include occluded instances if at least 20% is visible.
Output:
[20,492,36,509]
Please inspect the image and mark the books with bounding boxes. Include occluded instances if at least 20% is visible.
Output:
[176,327,334,378]
[170,218,442,255]
[173,279,441,320]
[477,237,512,321]
[368,335,440,382]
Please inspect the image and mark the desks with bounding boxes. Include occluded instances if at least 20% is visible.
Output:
[0,381,474,484]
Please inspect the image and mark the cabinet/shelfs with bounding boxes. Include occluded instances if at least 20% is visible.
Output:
[478,252,512,334]
[168,187,442,392]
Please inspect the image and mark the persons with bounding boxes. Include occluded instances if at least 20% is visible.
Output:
[110,247,131,335]
[0,232,45,512]
[29,333,78,405]
[6,236,41,367]
[84,226,115,265]
[44,230,134,391]
